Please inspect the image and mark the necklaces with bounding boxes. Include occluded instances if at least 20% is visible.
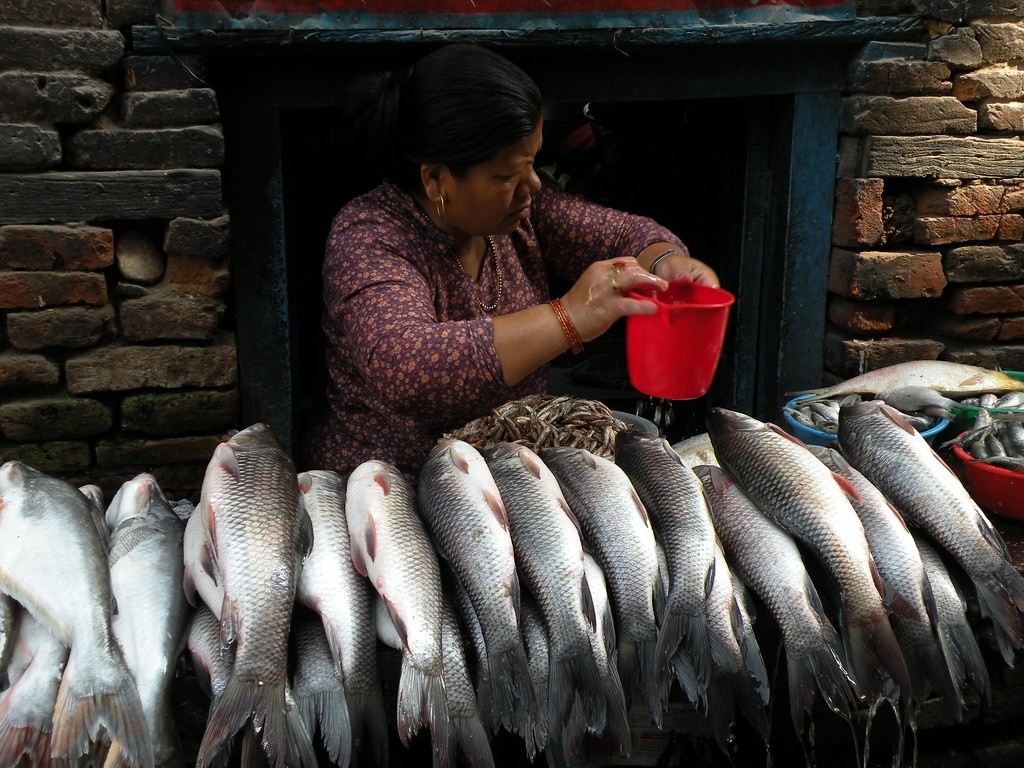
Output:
[421,212,503,310]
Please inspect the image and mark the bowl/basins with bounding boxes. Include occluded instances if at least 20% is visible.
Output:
[782,393,949,454]
[953,429,1024,520]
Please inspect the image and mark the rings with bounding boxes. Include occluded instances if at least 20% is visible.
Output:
[611,260,626,274]
[611,277,627,295]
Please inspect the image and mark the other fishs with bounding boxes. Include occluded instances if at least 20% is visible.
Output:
[0,359,1024,768]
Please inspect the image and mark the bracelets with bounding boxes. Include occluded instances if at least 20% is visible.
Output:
[548,297,585,356]
[648,248,678,275]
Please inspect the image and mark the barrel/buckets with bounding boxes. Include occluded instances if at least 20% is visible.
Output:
[625,279,736,401]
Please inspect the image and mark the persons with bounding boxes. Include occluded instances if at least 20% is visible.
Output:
[310,41,720,488]
